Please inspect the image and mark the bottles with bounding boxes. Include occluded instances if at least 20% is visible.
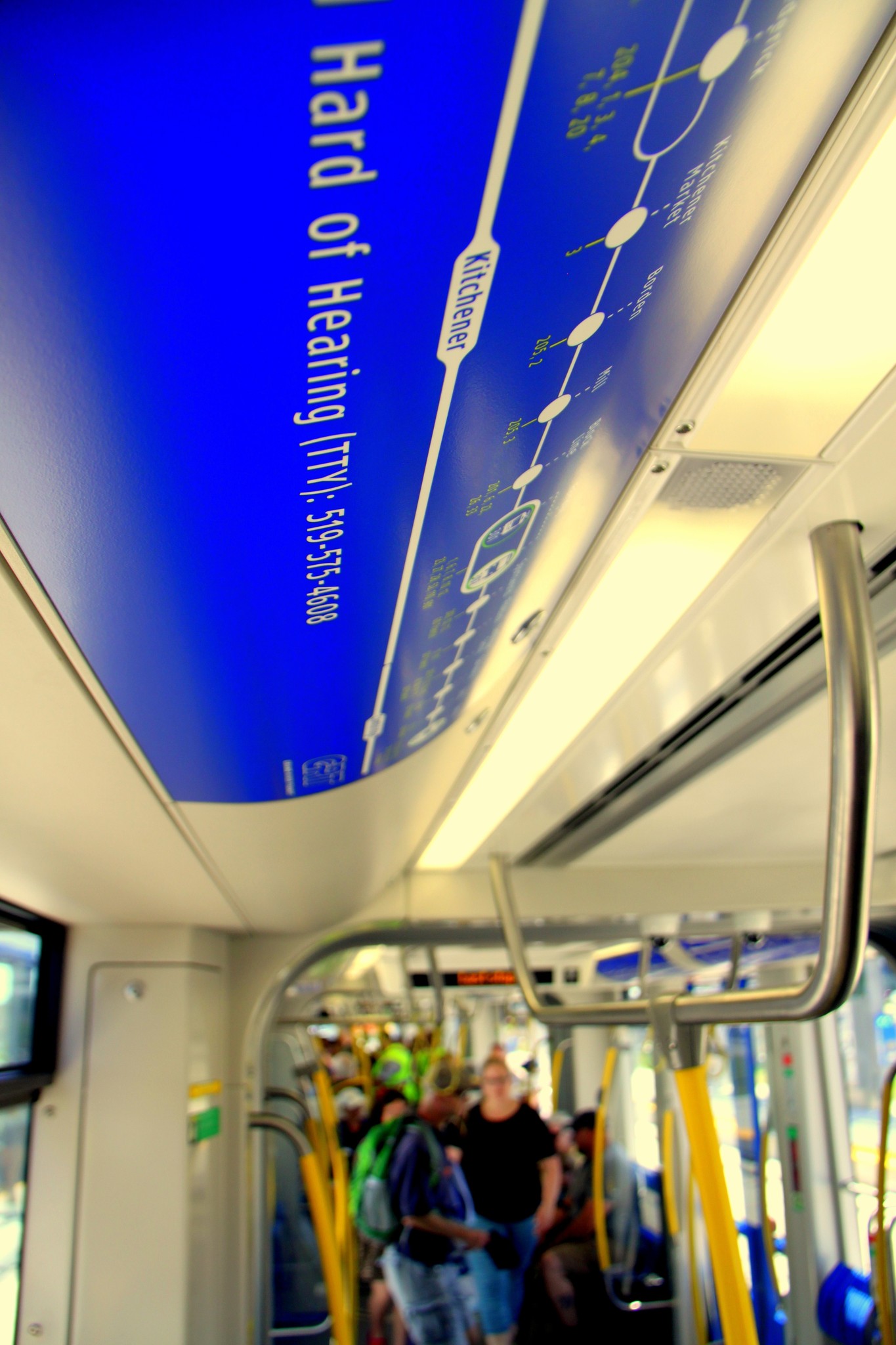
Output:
[366,1176,391,1229]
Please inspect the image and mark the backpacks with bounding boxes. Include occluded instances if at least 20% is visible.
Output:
[347,1127,401,1245]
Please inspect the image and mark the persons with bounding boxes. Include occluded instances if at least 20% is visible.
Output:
[309,1022,586,1189]
[446,1055,564,1335]
[542,1110,638,1327]
[373,1050,490,1345]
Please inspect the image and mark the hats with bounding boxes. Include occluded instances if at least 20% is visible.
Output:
[423,1059,483,1090]
